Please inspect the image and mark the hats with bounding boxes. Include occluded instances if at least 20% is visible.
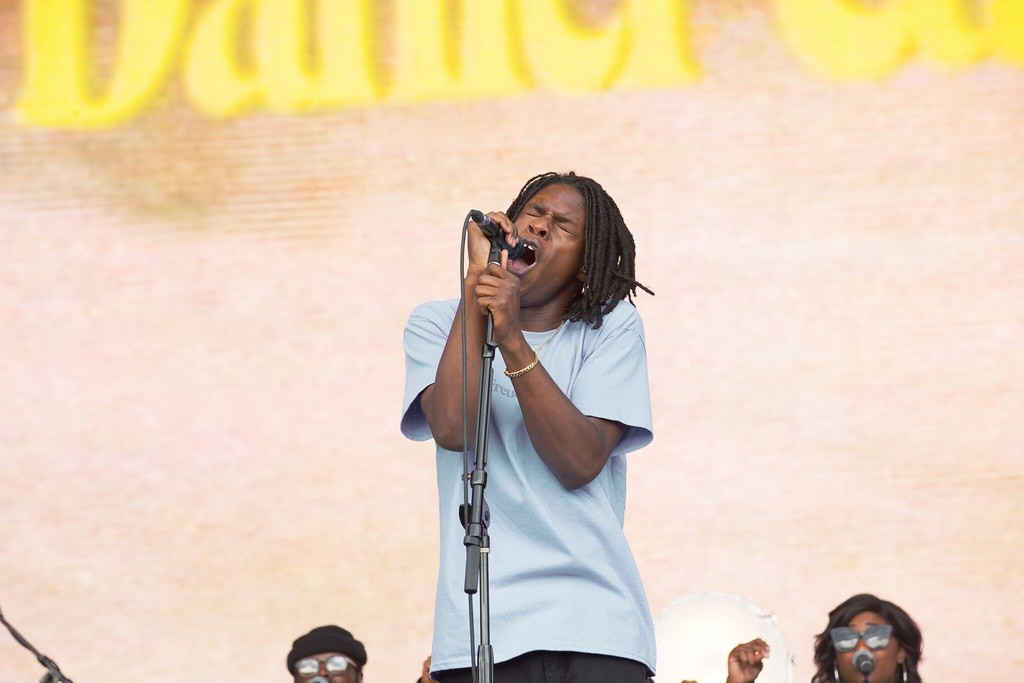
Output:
[286,625,367,677]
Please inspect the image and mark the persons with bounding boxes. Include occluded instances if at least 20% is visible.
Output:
[286,625,435,683]
[727,594,925,683]
[397,171,659,683]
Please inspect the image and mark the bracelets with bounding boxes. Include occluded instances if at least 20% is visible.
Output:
[504,351,538,377]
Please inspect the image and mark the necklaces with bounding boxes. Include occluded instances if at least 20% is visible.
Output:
[534,319,564,353]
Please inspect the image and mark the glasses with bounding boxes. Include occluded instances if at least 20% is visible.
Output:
[828,622,893,652]
[293,655,360,676]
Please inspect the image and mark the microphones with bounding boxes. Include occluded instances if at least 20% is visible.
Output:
[471,210,524,259]
[850,649,874,675]
